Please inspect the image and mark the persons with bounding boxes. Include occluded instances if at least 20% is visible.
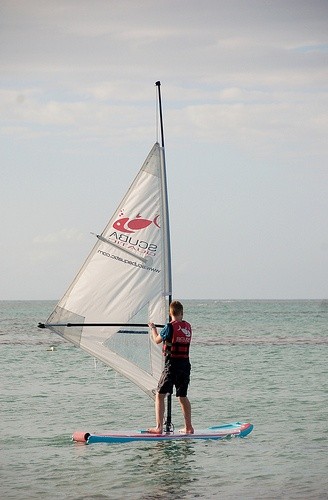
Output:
[147,300,194,437]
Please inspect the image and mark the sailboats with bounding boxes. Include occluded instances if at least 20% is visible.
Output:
[37,79,252,442]
